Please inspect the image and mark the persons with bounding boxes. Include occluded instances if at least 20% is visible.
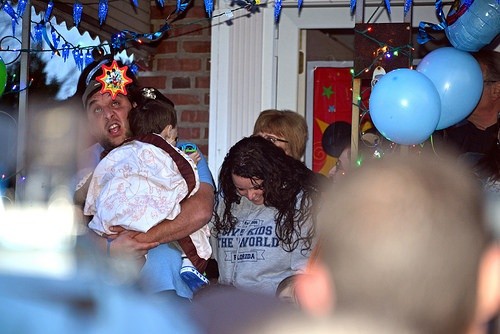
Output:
[73,75,217,305]
[205,135,329,303]
[293,142,500,334]
[437,50,500,187]
[0,54,135,224]
[253,109,309,161]
[74,85,214,296]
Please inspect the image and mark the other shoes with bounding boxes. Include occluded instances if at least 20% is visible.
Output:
[179,258,210,295]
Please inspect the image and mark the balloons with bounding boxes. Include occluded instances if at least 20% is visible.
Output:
[416,48,483,133]
[369,68,442,145]
[444,0,500,53]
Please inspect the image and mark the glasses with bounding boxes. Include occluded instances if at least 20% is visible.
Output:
[264,135,289,144]
